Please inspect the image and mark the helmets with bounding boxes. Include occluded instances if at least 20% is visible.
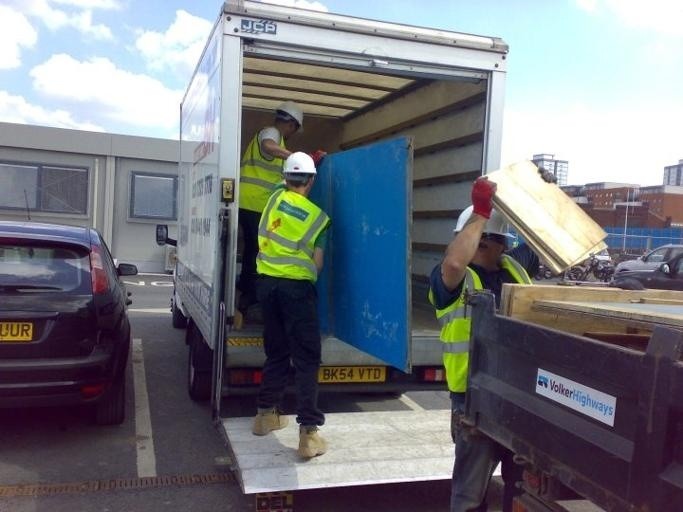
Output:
[279,151,317,174]
[450,202,518,240]
[273,101,305,134]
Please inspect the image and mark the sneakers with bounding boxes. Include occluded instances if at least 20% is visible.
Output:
[253,411,290,437]
[296,428,329,459]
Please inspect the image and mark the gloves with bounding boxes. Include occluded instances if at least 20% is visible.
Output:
[537,166,559,185]
[308,149,328,167]
[469,174,496,220]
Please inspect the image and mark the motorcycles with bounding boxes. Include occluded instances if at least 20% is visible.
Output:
[536,252,614,282]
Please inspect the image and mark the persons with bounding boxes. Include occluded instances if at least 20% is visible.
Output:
[249,149,332,460]
[236,98,327,335]
[424,166,576,512]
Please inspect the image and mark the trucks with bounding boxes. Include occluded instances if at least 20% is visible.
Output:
[155,0,510,495]
[451,289,683,512]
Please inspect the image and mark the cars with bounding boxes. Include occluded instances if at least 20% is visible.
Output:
[614,244,682,290]
[0,220,138,425]
